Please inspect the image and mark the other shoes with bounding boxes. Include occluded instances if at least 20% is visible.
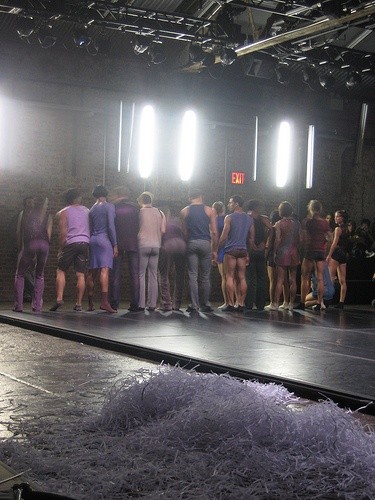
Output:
[73,305,82,311]
[264,303,344,311]
[217,303,243,311]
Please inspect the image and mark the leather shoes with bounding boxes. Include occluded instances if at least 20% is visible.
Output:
[100,304,117,313]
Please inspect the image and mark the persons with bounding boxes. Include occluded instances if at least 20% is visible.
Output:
[219,195,255,311]
[346,218,375,261]
[86,185,118,313]
[266,202,303,311]
[305,211,336,310]
[49,188,91,311]
[137,192,166,311]
[9,197,35,302]
[211,201,241,310]
[157,225,187,311]
[111,186,140,312]
[327,210,351,309]
[244,199,290,311]
[294,200,328,310]
[180,189,218,312]
[12,195,52,312]
